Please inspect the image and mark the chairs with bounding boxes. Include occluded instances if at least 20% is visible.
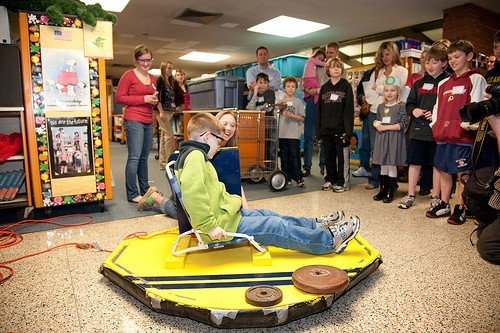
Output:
[165,160,268,255]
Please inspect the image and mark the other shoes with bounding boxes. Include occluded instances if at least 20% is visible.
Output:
[297,179,306,186]
[367,184,374,189]
[302,168,311,175]
[137,187,164,211]
[334,185,350,192]
[322,182,333,191]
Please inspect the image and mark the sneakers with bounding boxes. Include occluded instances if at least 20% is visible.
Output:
[447,204,467,225]
[319,210,346,229]
[431,195,439,206]
[326,215,360,253]
[398,195,415,209]
[425,201,452,218]
[352,166,372,177]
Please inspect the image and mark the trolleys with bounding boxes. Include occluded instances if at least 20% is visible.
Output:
[235,110,289,193]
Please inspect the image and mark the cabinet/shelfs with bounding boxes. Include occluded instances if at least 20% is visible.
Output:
[112,114,124,144]
[0,111,34,221]
[153,111,266,173]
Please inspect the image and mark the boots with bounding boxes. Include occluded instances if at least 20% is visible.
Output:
[373,174,389,201]
[384,176,398,202]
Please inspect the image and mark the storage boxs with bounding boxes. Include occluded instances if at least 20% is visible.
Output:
[187,77,246,108]
[216,54,309,77]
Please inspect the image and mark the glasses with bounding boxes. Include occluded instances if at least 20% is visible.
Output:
[137,58,155,63]
[200,132,225,146]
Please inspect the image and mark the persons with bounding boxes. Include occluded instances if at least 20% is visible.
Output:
[153,60,178,170]
[115,44,158,203]
[137,110,249,219]
[53,128,90,174]
[174,68,191,135]
[174,113,360,255]
[246,30,500,265]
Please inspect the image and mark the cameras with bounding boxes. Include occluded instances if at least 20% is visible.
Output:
[459,66,500,125]
